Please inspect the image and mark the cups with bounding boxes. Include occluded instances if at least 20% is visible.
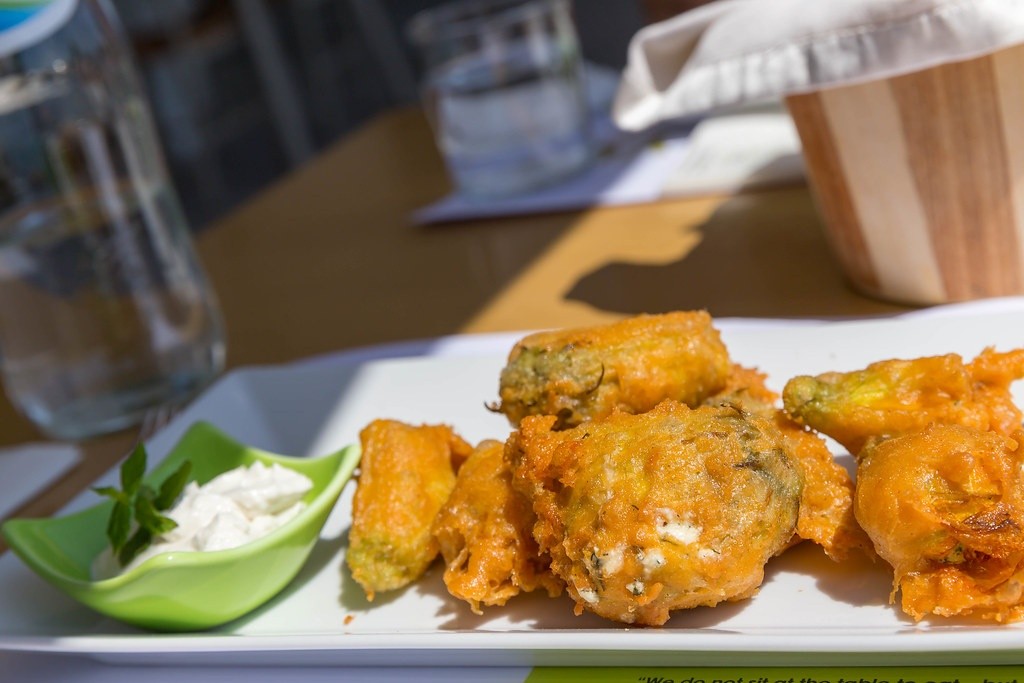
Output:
[406,1,591,195]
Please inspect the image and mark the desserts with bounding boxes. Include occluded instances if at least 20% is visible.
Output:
[91,460,312,581]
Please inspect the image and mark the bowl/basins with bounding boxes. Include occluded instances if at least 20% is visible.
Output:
[1,420,365,634]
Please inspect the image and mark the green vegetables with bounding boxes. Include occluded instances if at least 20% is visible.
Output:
[94,440,192,565]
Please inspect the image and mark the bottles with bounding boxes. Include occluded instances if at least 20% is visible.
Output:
[0,0,228,439]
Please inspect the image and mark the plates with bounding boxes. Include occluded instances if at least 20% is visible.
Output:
[1,294,1023,667]
